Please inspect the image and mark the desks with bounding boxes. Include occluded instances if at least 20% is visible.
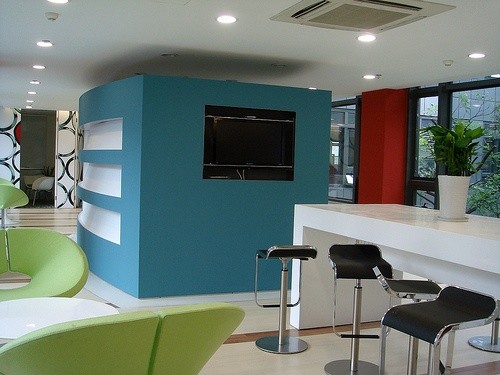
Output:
[290,204,500,329]
[0,297,118,346]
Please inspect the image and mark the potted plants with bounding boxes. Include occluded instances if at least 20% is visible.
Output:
[417,119,489,222]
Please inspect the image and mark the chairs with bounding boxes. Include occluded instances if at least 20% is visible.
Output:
[0,227,89,302]
[0,304,246,375]
[0,178,29,229]
[24,174,55,206]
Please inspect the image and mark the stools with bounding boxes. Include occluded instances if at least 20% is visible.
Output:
[372,266,500,375]
[255,245,317,354]
[324,243,394,375]
